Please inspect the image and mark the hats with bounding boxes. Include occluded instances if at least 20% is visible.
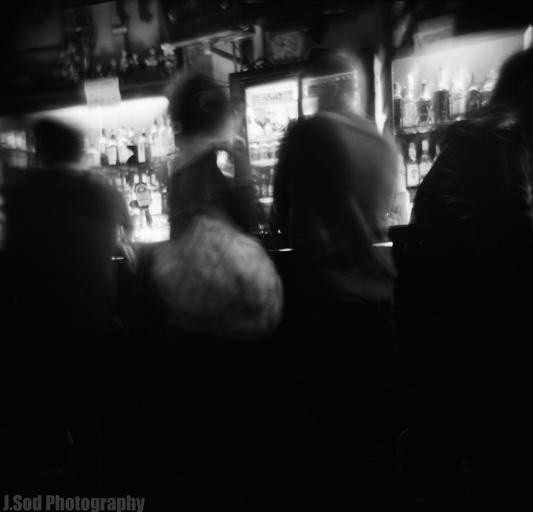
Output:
[154,215,284,336]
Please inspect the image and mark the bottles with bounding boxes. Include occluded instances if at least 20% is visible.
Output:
[390,65,502,191]
[87,114,175,215]
[67,41,178,89]
[250,168,275,199]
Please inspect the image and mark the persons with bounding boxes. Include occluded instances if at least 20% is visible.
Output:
[0,42,533,511]
